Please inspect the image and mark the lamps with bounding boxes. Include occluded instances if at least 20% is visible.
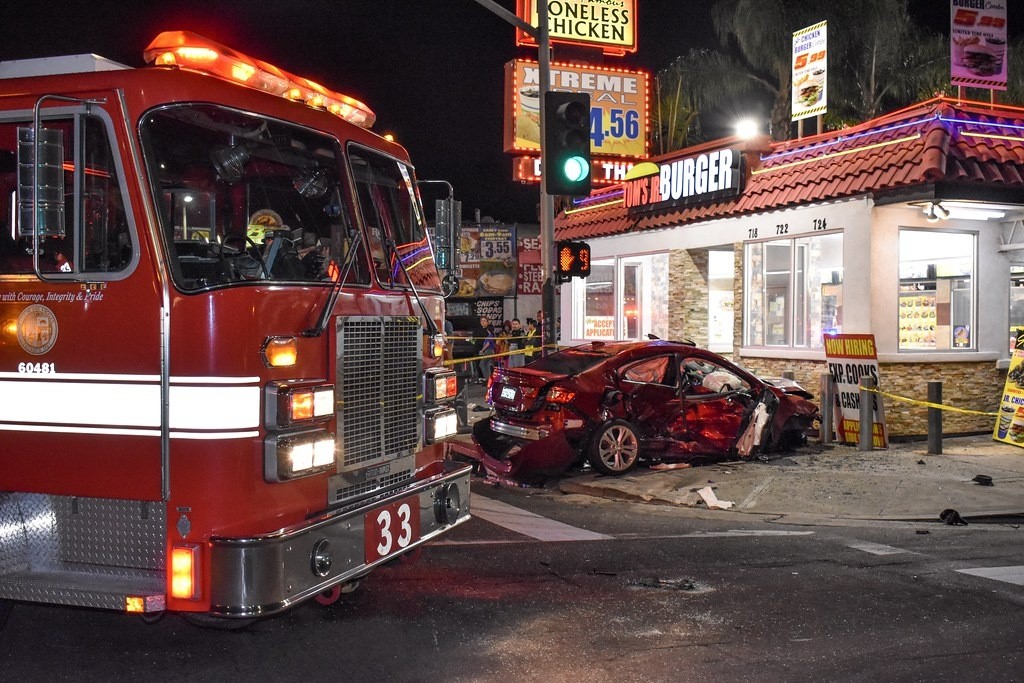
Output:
[926,204,950,222]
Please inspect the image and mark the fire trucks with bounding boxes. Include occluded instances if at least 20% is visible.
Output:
[0,27,474,632]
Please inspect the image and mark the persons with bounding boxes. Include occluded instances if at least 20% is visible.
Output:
[445,319,454,346]
[470,310,561,387]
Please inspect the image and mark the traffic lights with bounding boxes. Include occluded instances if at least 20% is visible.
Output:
[557,240,592,280]
[543,88,593,197]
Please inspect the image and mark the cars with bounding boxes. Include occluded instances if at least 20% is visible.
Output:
[472,332,822,485]
